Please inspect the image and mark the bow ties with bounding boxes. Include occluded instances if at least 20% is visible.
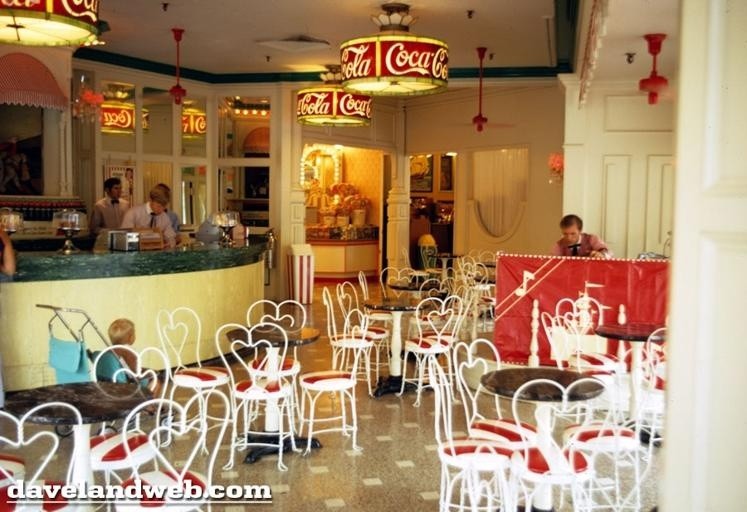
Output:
[111,199,119,204]
[150,212,156,228]
[568,244,581,256]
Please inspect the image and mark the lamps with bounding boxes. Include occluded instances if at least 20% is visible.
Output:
[180,100,206,140]
[2,0,104,49]
[335,2,452,96]
[293,64,371,127]
[101,90,150,136]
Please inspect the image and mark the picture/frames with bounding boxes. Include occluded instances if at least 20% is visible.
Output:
[410,155,433,193]
[438,153,455,193]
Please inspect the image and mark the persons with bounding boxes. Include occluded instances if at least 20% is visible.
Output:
[88,178,130,240]
[155,184,179,232]
[108,319,165,415]
[120,188,177,249]
[551,215,609,258]
[0,228,16,410]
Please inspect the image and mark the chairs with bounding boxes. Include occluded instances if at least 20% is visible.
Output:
[0,240,670,512]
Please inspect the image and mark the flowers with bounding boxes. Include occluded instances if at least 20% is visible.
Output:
[310,182,373,228]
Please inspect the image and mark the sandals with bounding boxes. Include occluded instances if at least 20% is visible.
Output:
[142,407,165,417]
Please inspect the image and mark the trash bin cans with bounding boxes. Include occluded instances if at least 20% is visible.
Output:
[286,244,316,305]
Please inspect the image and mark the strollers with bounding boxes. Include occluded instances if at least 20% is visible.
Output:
[34,302,176,441]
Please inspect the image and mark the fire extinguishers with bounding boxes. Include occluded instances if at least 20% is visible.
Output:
[265,227,276,270]
